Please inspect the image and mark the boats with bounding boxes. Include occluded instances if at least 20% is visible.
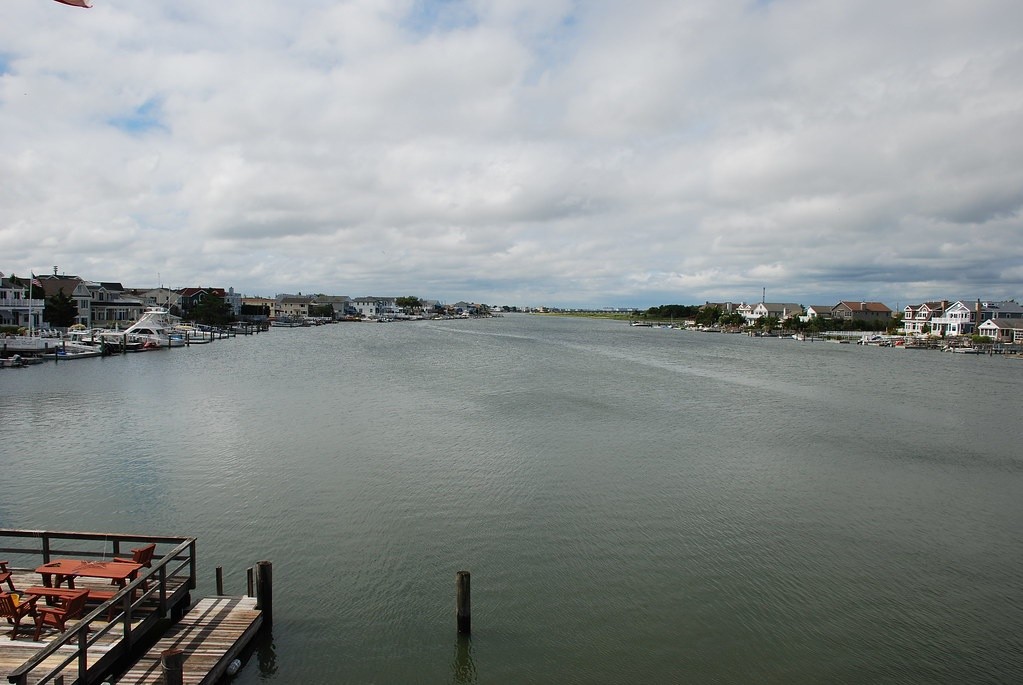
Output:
[879,343,896,348]
[950,346,978,353]
[941,348,950,352]
[0,354,42,367]
[176,319,319,341]
[102,271,183,347]
[631,324,652,327]
[839,341,850,344]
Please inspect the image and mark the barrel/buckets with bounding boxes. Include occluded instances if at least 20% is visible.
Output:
[2,593,20,607]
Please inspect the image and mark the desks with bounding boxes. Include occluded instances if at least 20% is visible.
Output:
[24,559,152,623]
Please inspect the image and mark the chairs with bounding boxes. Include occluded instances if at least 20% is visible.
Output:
[1,593,41,643]
[0,560,17,594]
[110,542,156,599]
[32,589,91,642]
